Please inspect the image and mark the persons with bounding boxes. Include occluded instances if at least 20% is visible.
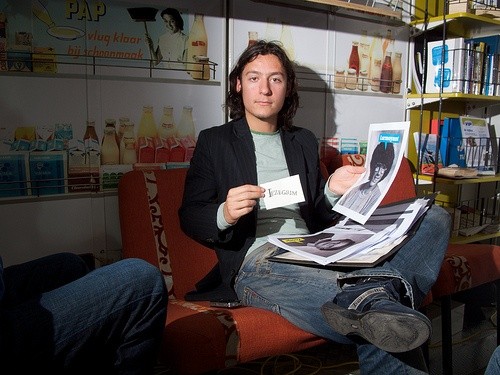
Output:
[339,142,395,216]
[144,8,189,70]
[0,252,168,375]
[278,232,354,251]
[177,40,452,375]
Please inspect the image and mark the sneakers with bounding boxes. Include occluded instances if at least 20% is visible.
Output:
[322,279,433,352]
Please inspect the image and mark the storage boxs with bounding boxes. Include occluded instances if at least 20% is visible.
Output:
[428,298,465,344]
[430,115,466,168]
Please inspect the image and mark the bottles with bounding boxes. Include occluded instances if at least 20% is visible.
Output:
[187,13,208,75]
[179,106,196,139]
[118,117,138,165]
[334,69,345,89]
[358,70,368,91]
[349,41,372,86]
[192,56,210,80]
[265,18,295,64]
[84,121,101,153]
[138,105,156,138]
[101,119,120,165]
[159,106,178,139]
[346,69,357,90]
[248,32,259,46]
[370,51,403,93]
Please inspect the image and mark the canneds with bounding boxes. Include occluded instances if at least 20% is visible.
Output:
[334,67,346,88]
[191,55,210,80]
[358,70,368,91]
[345,68,357,89]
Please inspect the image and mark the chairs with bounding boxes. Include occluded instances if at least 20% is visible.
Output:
[118,160,433,375]
[328,154,500,375]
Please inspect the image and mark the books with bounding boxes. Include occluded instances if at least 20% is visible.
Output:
[413,132,443,175]
[425,38,494,98]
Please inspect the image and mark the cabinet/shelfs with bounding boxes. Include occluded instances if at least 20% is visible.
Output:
[404,0,500,248]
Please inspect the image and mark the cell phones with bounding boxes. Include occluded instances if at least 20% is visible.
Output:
[209,297,242,307]
